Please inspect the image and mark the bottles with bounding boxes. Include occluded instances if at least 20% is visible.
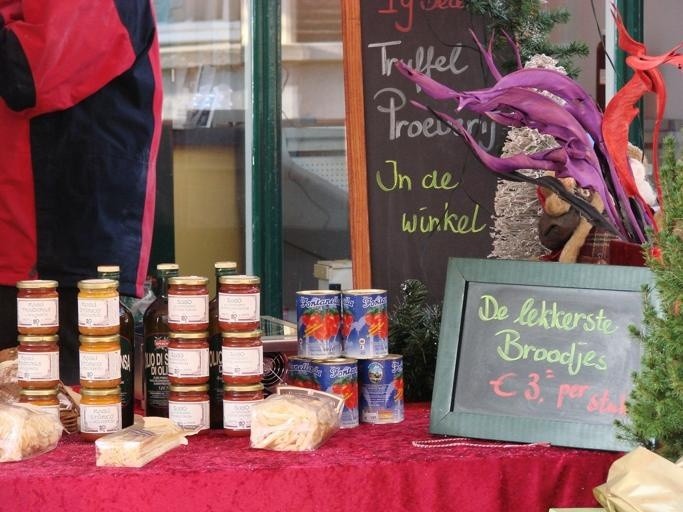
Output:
[142,260,181,413]
[96,260,133,432]
[206,262,234,428]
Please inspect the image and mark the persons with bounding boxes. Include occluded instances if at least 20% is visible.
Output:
[0,0,176,381]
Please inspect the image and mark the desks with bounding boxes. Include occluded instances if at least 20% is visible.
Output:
[0,402,631,510]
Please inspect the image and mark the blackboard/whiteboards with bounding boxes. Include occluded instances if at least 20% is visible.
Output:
[340,0,522,405]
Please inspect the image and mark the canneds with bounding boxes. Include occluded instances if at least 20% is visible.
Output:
[15,280,61,426]
[284,289,405,431]
[167,274,266,438]
[77,278,123,441]
